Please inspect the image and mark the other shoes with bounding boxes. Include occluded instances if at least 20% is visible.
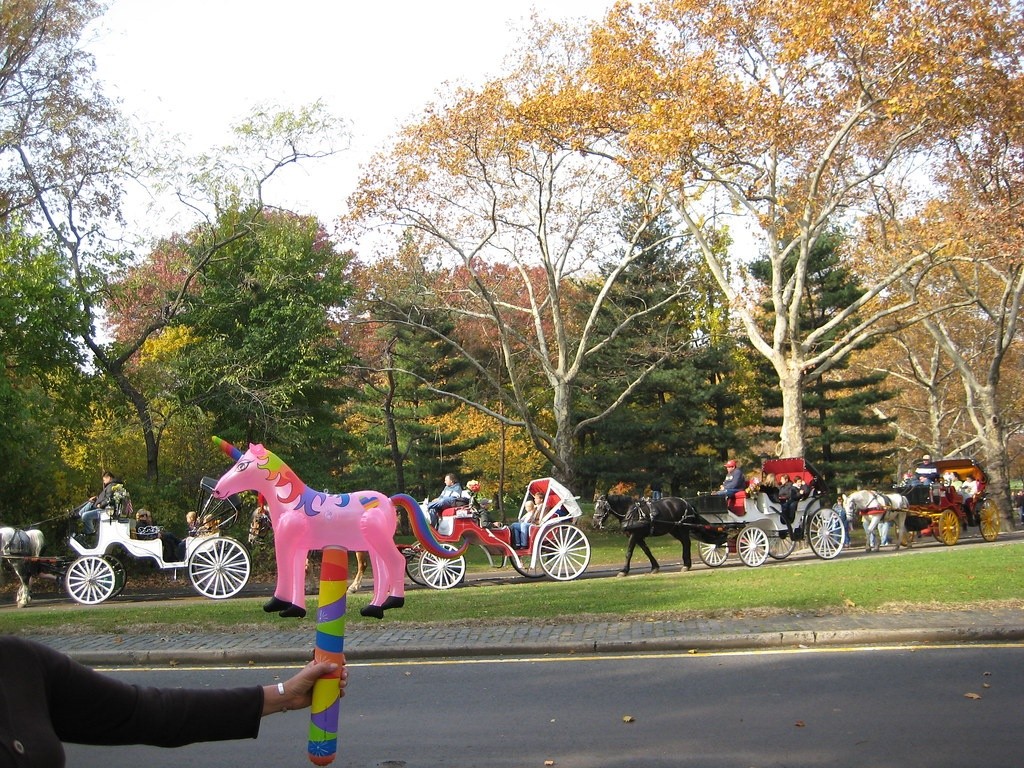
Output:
[880,543,888,546]
[513,546,521,550]
[845,544,849,548]
[81,529,98,536]
[520,546,527,549]
[870,543,874,548]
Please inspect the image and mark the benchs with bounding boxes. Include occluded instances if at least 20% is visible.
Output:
[131,526,167,556]
[796,490,820,512]
[965,481,984,510]
[473,507,511,547]
[730,481,751,498]
[185,520,221,553]
[529,512,559,541]
[942,486,963,503]
[757,492,783,514]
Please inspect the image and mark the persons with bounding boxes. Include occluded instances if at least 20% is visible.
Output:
[905,455,1024,538]
[135,508,218,563]
[479,491,550,550]
[68,471,119,537]
[711,460,746,498]
[0,634,349,768]
[760,473,809,525]
[832,497,891,546]
[429,473,464,529]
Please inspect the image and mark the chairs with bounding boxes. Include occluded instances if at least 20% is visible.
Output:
[931,478,945,489]
[436,489,475,516]
[98,502,123,520]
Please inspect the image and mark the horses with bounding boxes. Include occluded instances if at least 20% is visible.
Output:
[841,490,913,552]
[0,517,53,608]
[591,491,711,579]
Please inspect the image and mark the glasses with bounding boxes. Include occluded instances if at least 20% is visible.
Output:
[140,514,148,517]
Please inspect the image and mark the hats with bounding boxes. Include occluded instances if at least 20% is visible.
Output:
[724,460,736,467]
[923,455,931,459]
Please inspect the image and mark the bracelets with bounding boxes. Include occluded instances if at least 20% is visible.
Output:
[277,682,287,714]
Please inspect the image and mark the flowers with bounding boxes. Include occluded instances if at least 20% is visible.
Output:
[112,484,131,502]
[892,474,913,489]
[464,480,481,496]
[746,478,762,499]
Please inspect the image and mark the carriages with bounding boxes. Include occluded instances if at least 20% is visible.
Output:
[1,476,254,609]
[248,477,592,590]
[594,456,846,578]
[842,457,1001,554]
